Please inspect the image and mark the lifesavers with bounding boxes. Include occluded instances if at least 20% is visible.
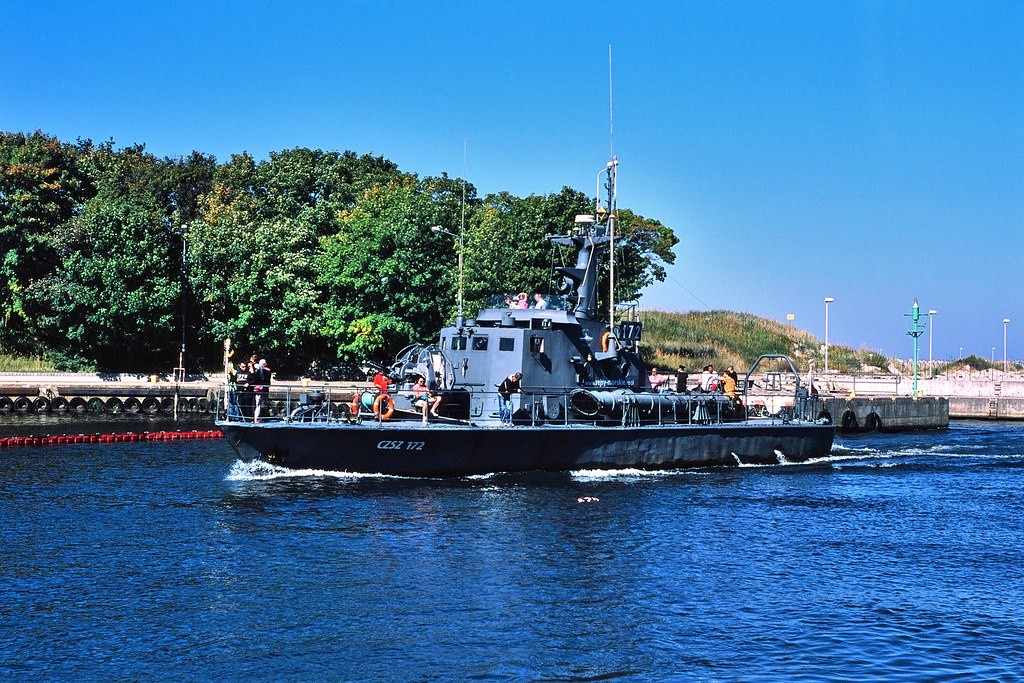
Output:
[32,396,51,416]
[87,398,105,414]
[142,396,161,417]
[866,412,883,431]
[106,398,124,416]
[177,398,188,412]
[197,399,224,413]
[69,397,87,419]
[602,331,618,351]
[13,396,32,415]
[161,398,174,414]
[124,397,142,415]
[267,401,350,416]
[51,397,68,416]
[0,396,13,415]
[352,393,361,414]
[188,398,198,412]
[818,410,832,424]
[373,394,395,420]
[842,409,857,431]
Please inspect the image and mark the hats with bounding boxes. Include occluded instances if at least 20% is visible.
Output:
[259,359,267,367]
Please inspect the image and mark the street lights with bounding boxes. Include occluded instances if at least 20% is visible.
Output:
[824,297,835,374]
[1003,319,1011,371]
[928,309,938,378]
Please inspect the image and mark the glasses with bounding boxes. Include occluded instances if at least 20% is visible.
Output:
[420,381,424,383]
[241,365,246,368]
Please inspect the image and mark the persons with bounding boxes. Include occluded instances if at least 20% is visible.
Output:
[676,365,689,392]
[374,372,392,393]
[798,385,818,419]
[699,365,737,398]
[498,372,522,426]
[649,368,664,388]
[505,293,528,309]
[413,377,441,424]
[229,355,272,423]
[534,292,546,310]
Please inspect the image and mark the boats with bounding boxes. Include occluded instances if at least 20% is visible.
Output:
[213,42,838,479]
